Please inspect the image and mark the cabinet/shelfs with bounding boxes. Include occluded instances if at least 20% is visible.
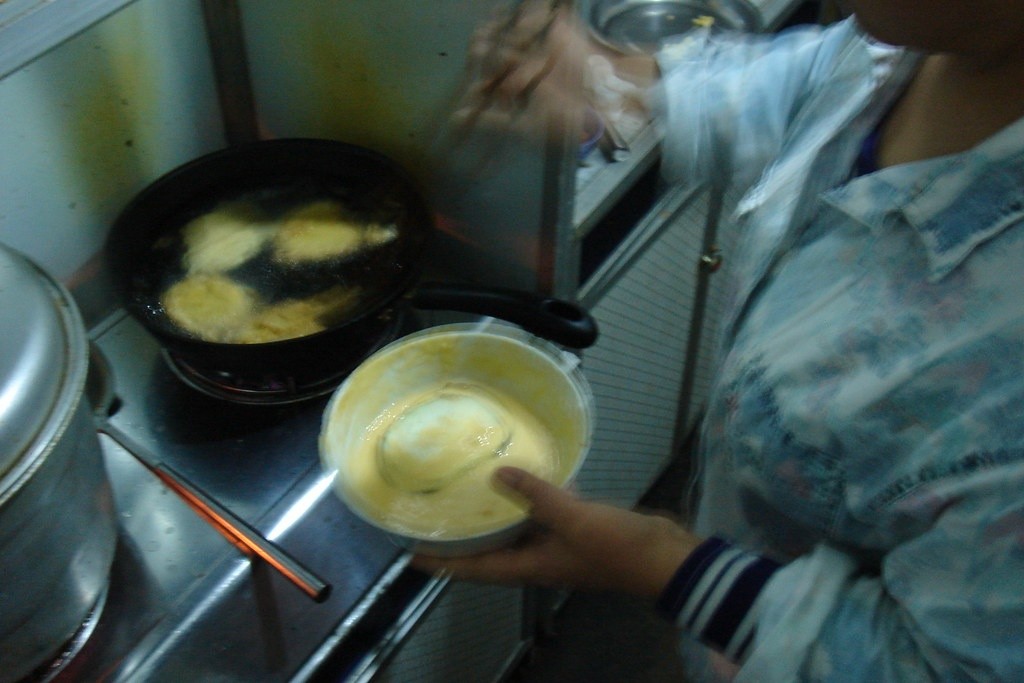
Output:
[349,172,727,683]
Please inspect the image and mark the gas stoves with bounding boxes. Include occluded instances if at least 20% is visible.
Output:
[35,305,498,682]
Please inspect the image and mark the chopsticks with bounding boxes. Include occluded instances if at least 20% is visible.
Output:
[412,0,565,191]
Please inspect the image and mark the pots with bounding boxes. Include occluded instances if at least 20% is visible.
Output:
[0,242,118,683]
[107,137,600,371]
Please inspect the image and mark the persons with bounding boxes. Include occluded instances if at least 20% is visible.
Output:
[412,0,1024,683]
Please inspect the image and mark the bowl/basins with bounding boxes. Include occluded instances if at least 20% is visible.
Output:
[579,1,764,56]
[579,109,603,162]
[318,322,597,559]
